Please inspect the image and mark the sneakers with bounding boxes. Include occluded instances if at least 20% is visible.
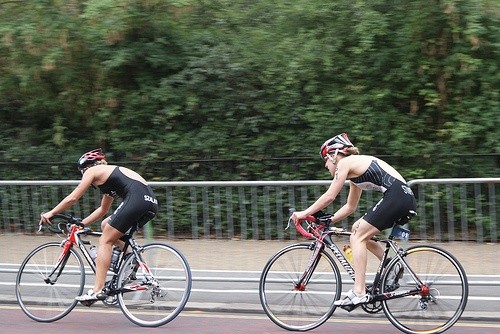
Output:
[385,262,404,291]
[75,289,105,301]
[334,290,367,305]
[127,254,142,281]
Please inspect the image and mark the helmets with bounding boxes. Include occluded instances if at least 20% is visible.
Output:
[319,133,354,160]
[77,148,106,171]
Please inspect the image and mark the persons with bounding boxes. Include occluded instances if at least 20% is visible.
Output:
[292,133,416,307]
[38,150,159,301]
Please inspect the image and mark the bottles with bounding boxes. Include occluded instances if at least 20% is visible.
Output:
[342,245,352,263]
[89,245,97,264]
[109,246,121,271]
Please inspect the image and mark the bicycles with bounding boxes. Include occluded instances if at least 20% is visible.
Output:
[259,208,469,334]
[16,212,192,327]
